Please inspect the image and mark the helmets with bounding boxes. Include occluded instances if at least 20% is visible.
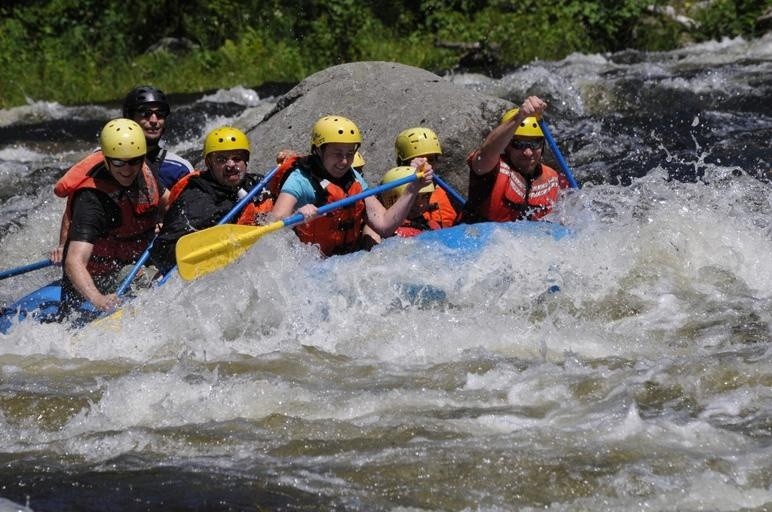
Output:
[311,115,442,205]
[124,86,170,121]
[501,108,545,138]
[203,127,249,159]
[100,118,147,170]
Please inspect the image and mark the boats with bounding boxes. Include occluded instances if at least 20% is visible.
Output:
[0,200,574,364]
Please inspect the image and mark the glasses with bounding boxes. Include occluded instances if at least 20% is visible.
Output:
[510,139,542,150]
[136,110,168,119]
[111,159,142,167]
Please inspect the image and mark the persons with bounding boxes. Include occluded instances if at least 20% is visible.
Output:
[265,115,434,258]
[346,144,389,250]
[395,128,459,226]
[46,87,193,273]
[374,166,442,239]
[51,119,172,313]
[144,126,302,292]
[454,94,571,228]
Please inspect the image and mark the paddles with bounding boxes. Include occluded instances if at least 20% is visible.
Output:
[88,162,288,335]
[174,170,426,283]
